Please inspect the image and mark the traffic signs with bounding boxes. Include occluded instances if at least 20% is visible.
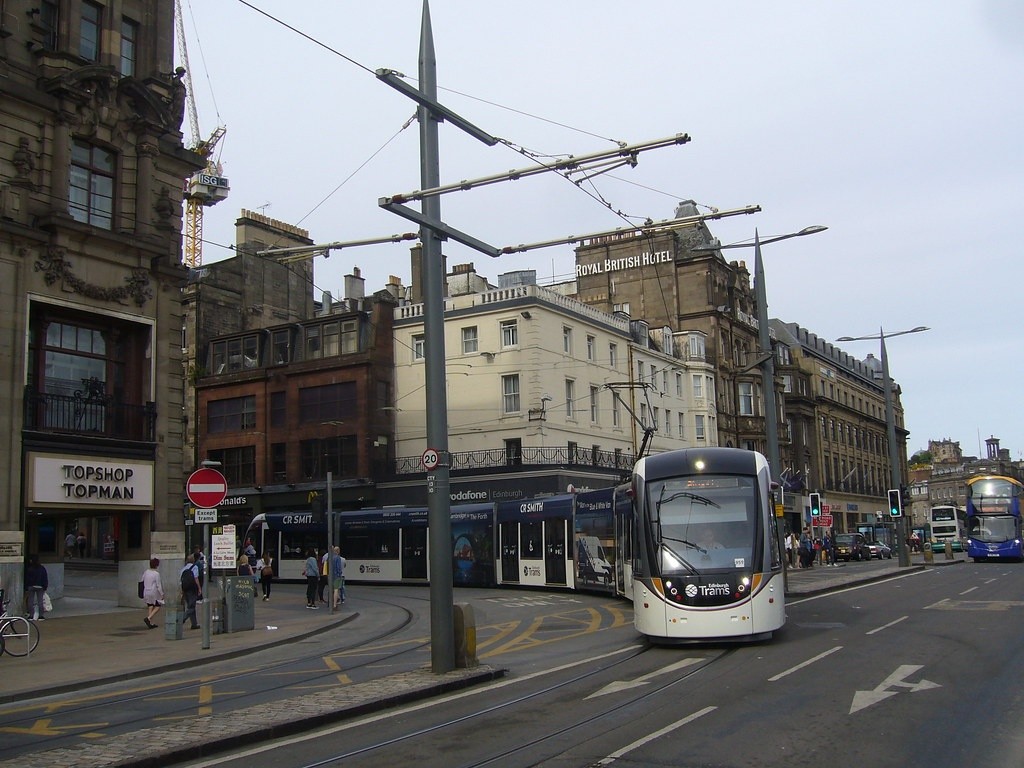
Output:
[212,534,236,569]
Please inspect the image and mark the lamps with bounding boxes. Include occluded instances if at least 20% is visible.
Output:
[521,310,531,318]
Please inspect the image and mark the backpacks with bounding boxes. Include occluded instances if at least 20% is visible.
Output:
[181,564,199,594]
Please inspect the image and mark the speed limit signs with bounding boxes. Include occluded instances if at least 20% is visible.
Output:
[421,449,439,469]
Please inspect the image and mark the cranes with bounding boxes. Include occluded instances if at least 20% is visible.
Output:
[174,0,230,269]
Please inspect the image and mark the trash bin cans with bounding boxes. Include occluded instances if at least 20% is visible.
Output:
[218,577,255,633]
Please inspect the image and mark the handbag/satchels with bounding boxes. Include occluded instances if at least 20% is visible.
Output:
[138,581,144,599]
[323,557,329,575]
[43,592,52,612]
[262,566,272,575]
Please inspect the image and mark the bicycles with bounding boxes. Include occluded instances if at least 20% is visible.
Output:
[0,599,41,658]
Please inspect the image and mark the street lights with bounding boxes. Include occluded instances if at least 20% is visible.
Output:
[200,458,222,648]
[688,224,828,592]
[836,325,933,568]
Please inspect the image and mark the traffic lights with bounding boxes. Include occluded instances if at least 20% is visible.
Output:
[809,493,821,517]
[888,489,902,517]
[900,484,909,507]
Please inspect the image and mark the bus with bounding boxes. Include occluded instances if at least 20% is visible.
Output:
[965,475,1024,563]
[930,505,968,553]
[241,380,785,643]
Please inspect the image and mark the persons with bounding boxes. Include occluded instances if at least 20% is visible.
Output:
[64,529,76,559]
[784,523,840,570]
[238,539,276,602]
[77,532,86,559]
[300,544,347,610]
[22,553,49,622]
[912,540,919,555]
[103,533,110,551]
[140,558,165,629]
[181,545,214,630]
[693,527,724,550]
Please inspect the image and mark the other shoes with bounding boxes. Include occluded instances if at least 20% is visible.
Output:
[37,616,45,621]
[263,595,266,601]
[144,617,152,628]
[191,624,200,629]
[148,623,158,629]
[204,597,209,602]
[265,598,270,601]
[196,600,202,604]
[306,603,320,609]
[27,616,33,620]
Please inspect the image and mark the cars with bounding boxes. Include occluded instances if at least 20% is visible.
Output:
[835,533,871,561]
[866,540,893,560]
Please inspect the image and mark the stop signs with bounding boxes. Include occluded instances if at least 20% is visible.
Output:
[186,468,229,510]
